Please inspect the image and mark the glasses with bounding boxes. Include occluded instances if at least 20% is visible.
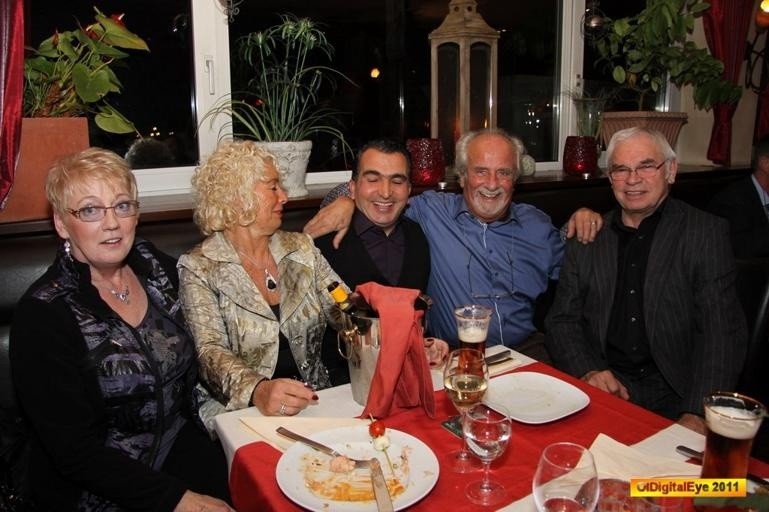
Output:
[468,250,513,298]
[610,160,665,182]
[65,200,139,222]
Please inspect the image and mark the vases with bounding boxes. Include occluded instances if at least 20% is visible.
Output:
[400,138,446,189]
[561,132,600,181]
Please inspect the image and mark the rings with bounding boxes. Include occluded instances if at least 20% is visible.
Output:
[591,223,596,225]
[279,404,288,415]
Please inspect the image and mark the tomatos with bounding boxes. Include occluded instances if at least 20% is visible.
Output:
[368,419,386,437]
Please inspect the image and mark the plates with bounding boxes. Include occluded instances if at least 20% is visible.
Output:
[480,369,591,425]
[275,426,438,512]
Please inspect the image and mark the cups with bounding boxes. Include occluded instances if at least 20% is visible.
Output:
[631,484,687,512]
[453,302,492,349]
[698,388,763,479]
[529,442,599,511]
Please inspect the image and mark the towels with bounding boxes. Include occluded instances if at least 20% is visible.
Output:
[432,353,523,382]
[235,413,378,456]
[571,430,703,484]
[351,281,436,424]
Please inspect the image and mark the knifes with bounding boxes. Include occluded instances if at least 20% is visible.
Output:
[677,444,769,489]
[370,457,394,512]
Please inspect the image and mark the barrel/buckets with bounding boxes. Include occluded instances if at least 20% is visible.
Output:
[336,293,428,407]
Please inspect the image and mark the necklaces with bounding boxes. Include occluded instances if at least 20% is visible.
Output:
[239,248,277,292]
[107,285,133,305]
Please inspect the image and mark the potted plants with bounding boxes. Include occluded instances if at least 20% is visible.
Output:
[0,5,147,225]
[578,2,742,155]
[199,10,359,193]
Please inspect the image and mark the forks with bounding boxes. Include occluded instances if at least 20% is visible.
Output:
[276,426,371,468]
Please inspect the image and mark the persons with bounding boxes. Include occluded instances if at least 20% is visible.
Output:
[717,138,768,314]
[8,147,235,511]
[545,127,749,434]
[304,129,603,380]
[313,136,430,387]
[176,144,452,443]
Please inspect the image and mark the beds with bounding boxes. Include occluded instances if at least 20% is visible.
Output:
[202,336,768,511]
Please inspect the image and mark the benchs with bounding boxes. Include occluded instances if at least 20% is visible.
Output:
[0,167,759,510]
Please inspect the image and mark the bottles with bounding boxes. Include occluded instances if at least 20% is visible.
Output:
[405,137,446,187]
[563,135,597,176]
[327,279,356,314]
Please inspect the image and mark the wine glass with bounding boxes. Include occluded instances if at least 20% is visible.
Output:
[442,349,491,474]
[462,402,512,506]
